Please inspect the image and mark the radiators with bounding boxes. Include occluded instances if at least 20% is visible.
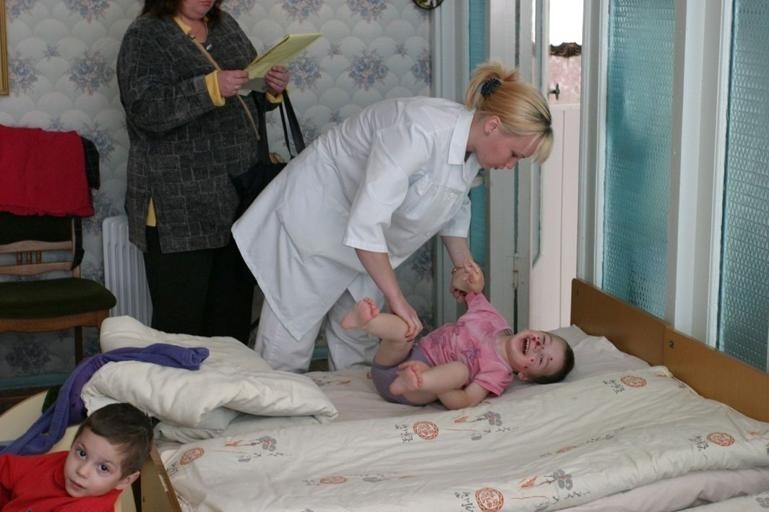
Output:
[100,215,156,328]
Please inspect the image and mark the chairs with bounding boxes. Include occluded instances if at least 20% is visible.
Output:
[2,212,120,366]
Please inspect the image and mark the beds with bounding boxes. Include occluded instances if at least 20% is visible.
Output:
[135,275,768,511]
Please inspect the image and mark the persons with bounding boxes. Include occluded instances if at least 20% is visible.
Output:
[0,400,156,512]
[225,53,557,376]
[336,260,579,410]
[115,0,289,347]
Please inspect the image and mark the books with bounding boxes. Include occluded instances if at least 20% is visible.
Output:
[237,27,321,95]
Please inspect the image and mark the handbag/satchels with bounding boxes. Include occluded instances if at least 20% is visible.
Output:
[227,159,294,290]
